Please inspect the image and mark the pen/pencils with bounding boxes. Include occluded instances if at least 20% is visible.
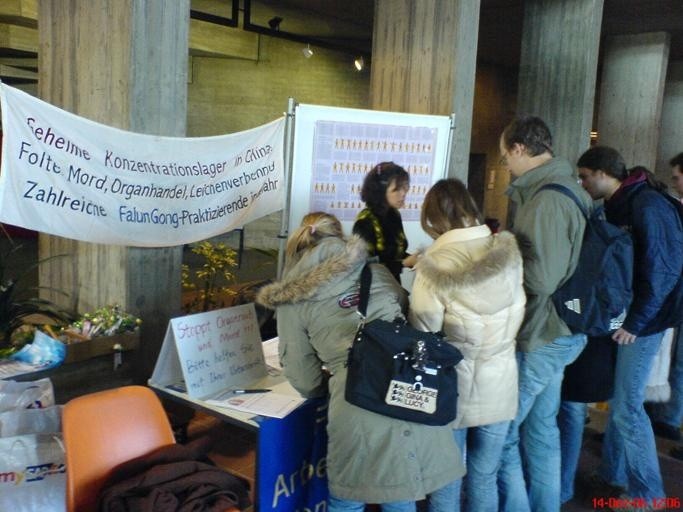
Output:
[233,389,272,394]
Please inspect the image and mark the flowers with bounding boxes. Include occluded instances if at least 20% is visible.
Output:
[56,305,137,345]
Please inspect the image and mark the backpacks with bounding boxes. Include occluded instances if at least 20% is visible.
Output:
[552,208,636,337]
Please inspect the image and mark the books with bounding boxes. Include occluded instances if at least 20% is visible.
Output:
[205,385,308,419]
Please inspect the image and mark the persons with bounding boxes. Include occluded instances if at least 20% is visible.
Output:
[408,177,528,511]
[257,213,467,511]
[353,161,421,283]
[556,399,587,511]
[572,145,680,511]
[653,151,683,458]
[497,115,596,511]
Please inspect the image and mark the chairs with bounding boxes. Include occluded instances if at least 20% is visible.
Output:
[60,384,243,512]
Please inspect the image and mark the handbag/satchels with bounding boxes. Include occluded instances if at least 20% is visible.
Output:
[562,338,617,403]
[344,319,462,426]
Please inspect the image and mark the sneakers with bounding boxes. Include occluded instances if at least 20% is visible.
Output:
[652,421,679,440]
[577,479,624,507]
